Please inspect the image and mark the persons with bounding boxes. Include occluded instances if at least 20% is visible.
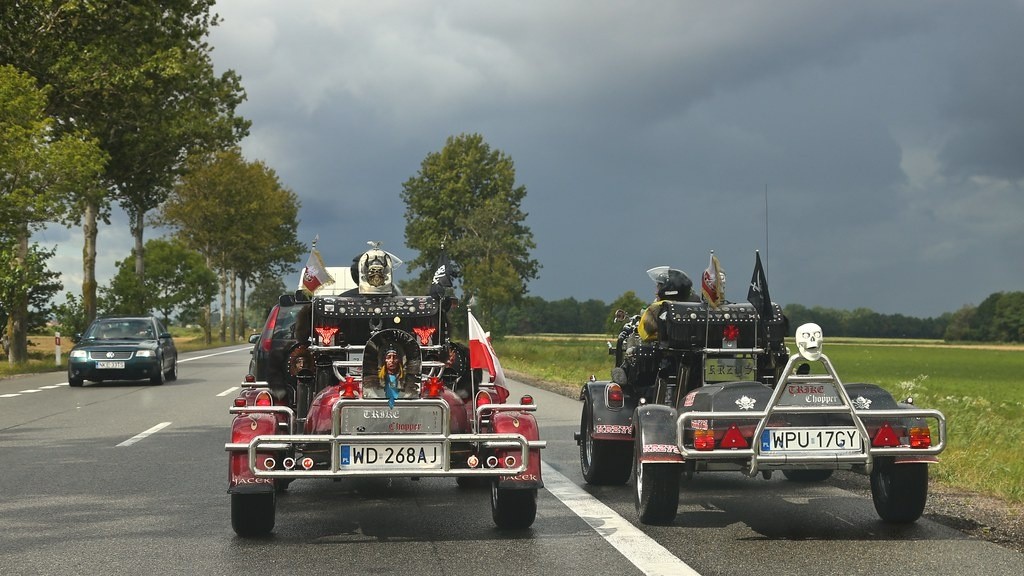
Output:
[342,251,403,296]
[647,265,692,302]
[138,322,153,336]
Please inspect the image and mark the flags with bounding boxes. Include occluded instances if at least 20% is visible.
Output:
[301,251,335,294]
[702,255,722,306]
[468,313,509,402]
[748,253,773,322]
[431,250,452,300]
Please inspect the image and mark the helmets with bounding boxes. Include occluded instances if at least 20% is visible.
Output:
[351,254,365,285]
[657,270,692,301]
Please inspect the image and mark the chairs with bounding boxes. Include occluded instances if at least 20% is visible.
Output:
[107,328,121,338]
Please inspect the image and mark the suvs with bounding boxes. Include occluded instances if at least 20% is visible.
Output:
[247,302,314,416]
[573,179,950,526]
[224,229,546,538]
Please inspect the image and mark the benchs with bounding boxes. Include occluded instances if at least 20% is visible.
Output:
[308,294,451,359]
[632,301,790,386]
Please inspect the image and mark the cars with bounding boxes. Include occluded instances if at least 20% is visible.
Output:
[68,317,178,387]
